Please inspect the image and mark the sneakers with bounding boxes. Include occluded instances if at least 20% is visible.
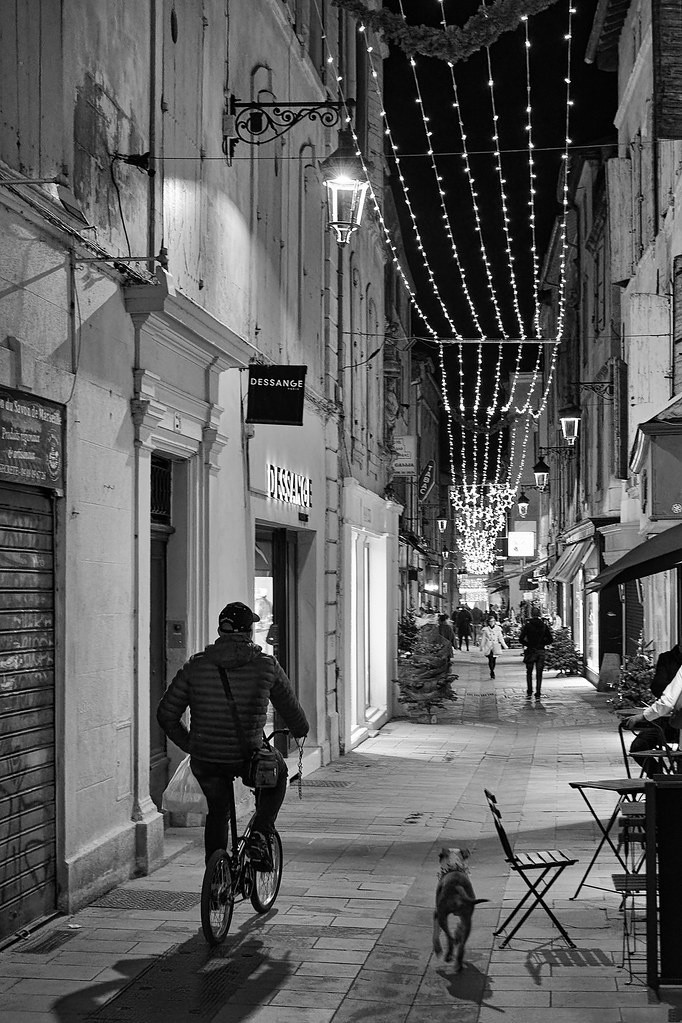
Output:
[210,891,227,909]
[245,831,273,872]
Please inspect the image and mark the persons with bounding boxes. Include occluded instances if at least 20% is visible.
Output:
[156,602,309,910]
[484,604,516,624]
[456,605,472,651]
[414,603,472,629]
[630,643,682,777]
[437,613,458,658]
[519,608,554,699]
[479,616,508,680]
[623,664,682,753]
[471,603,484,647]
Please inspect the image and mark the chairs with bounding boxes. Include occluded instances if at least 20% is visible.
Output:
[614,802,647,857]
[611,818,661,985]
[484,789,580,950]
[619,718,682,782]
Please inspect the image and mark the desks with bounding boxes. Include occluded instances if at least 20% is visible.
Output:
[569,778,649,912]
[616,709,678,719]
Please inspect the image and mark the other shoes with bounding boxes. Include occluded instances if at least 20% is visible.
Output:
[529,690,532,696]
[536,695,540,700]
[490,671,495,679]
[458,647,461,650]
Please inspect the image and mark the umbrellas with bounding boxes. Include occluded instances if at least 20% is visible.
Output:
[584,522,682,594]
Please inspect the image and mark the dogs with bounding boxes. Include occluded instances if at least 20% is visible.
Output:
[433,846,493,975]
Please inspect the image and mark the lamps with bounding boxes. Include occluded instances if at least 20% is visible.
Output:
[518,483,549,519]
[532,446,578,491]
[439,540,449,561]
[421,504,449,534]
[222,93,372,249]
[557,378,614,446]
[394,339,417,351]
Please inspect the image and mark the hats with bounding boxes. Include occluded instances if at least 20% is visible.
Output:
[219,602,260,634]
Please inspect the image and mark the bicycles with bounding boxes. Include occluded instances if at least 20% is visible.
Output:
[200,729,308,949]
[471,625,479,645]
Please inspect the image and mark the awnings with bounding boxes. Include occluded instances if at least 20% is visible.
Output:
[483,555,554,594]
[547,540,595,584]
[423,590,446,599]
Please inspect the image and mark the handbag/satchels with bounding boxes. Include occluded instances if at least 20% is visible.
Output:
[243,749,279,788]
[523,648,538,664]
[162,754,210,815]
[493,643,503,655]
